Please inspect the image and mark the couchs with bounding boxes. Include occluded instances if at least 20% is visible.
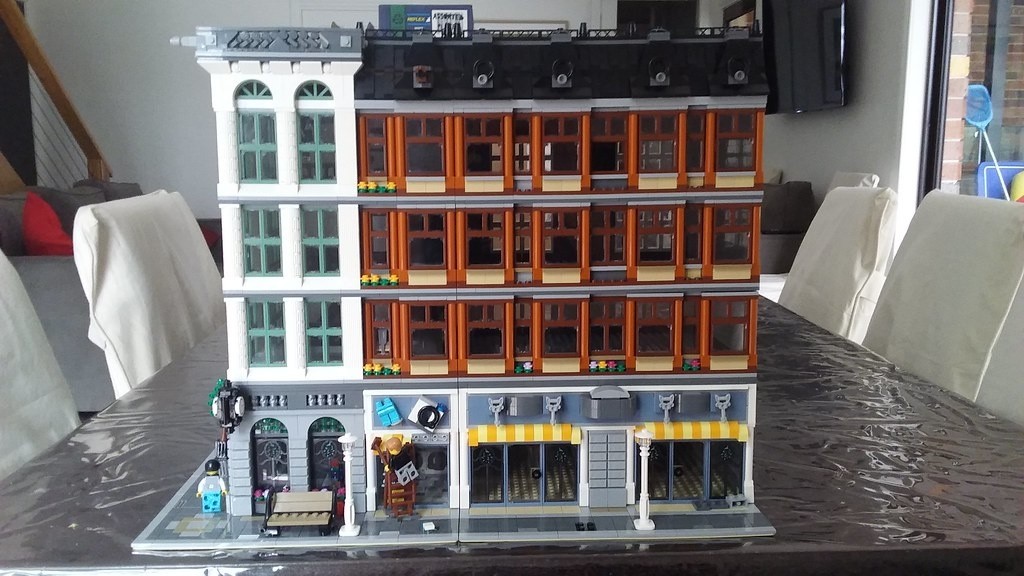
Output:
[760,165,815,299]
[0,177,222,413]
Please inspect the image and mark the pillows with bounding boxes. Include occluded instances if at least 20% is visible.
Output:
[202,227,219,248]
[23,192,75,256]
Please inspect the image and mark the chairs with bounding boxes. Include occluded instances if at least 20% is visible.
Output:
[0,246,83,490]
[71,190,229,399]
[864,189,1023,403]
[778,168,900,343]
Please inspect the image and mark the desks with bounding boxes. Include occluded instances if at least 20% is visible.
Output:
[1,295,1024,576]
